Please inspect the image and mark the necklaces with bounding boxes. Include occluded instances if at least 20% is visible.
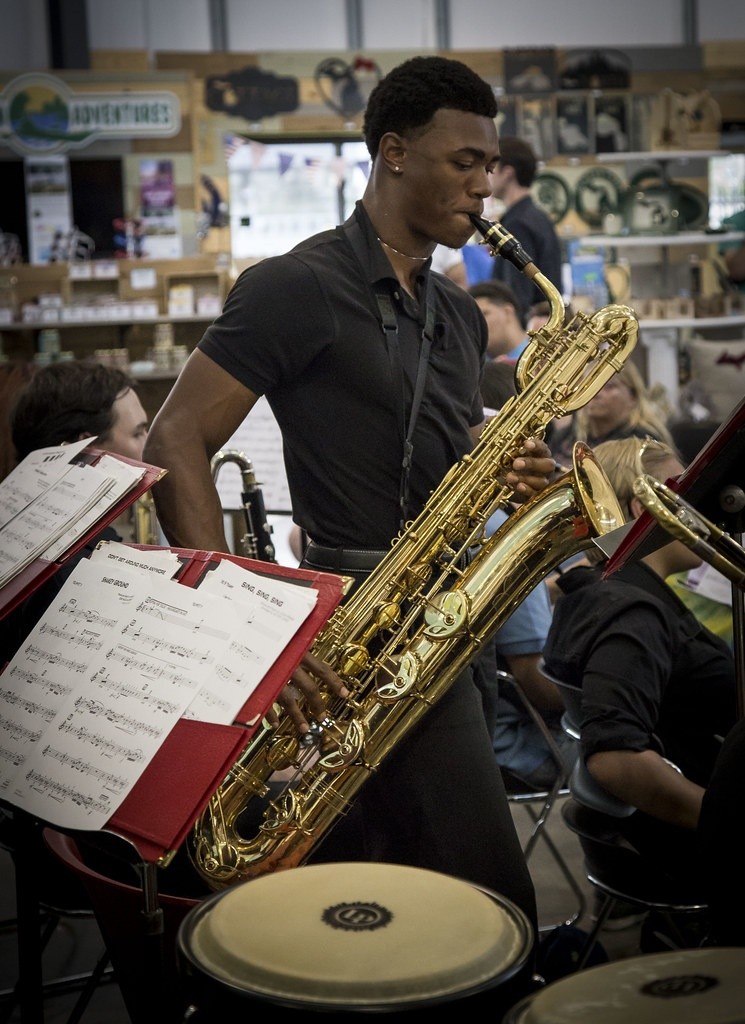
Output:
[376,233,433,263]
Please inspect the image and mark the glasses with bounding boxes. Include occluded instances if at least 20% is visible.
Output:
[638,432,666,460]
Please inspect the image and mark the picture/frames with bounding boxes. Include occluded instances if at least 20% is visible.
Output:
[478,36,635,164]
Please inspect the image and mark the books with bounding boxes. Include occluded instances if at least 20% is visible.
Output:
[1,437,169,626]
[0,541,354,868]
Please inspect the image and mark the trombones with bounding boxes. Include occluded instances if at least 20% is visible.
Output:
[632,474,745,593]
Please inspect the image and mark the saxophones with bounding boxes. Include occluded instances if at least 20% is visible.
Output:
[185,215,641,897]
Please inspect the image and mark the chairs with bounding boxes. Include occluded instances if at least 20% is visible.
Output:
[492,660,586,936]
[43,827,206,1024]
[519,702,713,967]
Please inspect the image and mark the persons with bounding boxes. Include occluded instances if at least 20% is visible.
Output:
[463,276,532,363]
[568,354,681,453]
[1,362,209,1024]
[143,54,557,1000]
[472,360,583,790]
[523,293,579,427]
[543,435,745,951]
[487,133,563,308]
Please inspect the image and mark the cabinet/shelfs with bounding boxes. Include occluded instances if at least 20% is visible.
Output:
[0,252,228,399]
[578,227,745,330]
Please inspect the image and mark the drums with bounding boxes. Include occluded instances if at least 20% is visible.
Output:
[176,860,532,1024]
[504,948,745,1024]
